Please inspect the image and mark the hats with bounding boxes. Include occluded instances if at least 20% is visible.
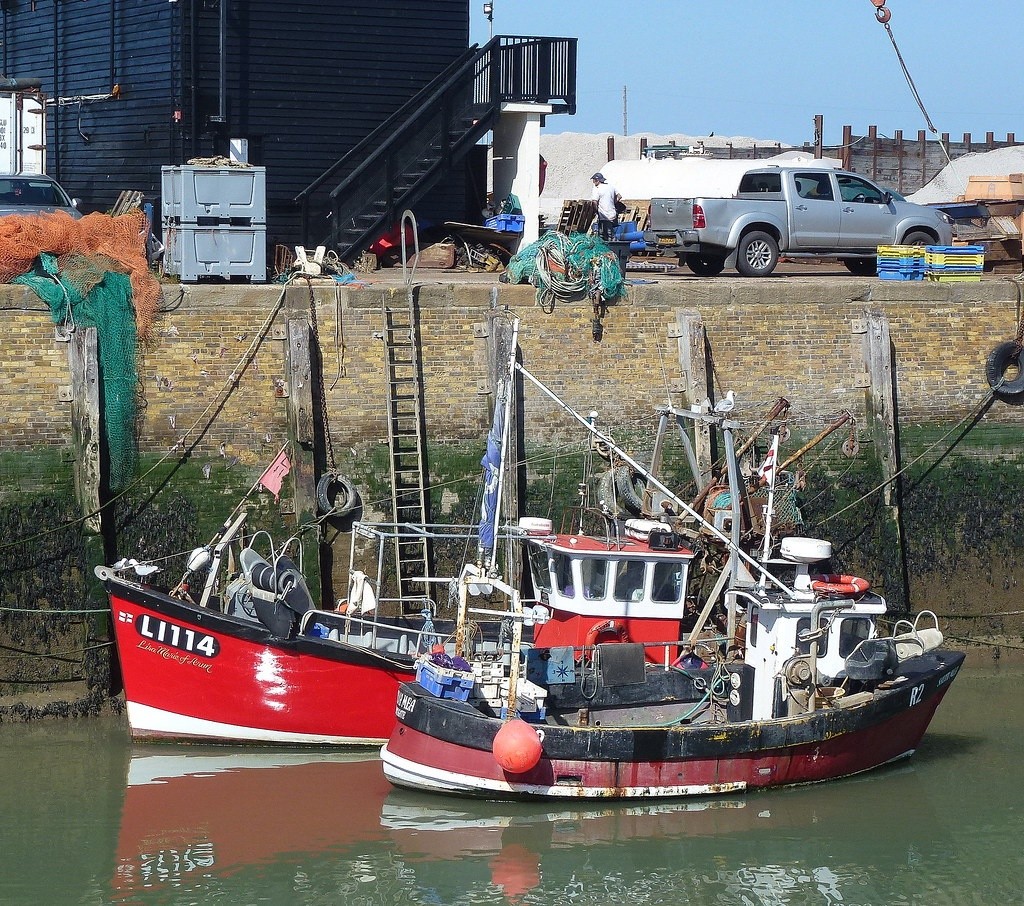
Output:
[593,173,606,182]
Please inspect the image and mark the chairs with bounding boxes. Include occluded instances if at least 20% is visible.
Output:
[795,180,802,193]
[815,180,833,201]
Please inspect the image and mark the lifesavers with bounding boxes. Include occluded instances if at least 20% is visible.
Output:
[583,619,630,662]
[595,465,650,521]
[809,573,871,594]
[315,471,358,517]
[985,341,1024,394]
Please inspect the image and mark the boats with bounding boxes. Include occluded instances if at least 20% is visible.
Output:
[380,313,966,803]
[105,743,385,899]
[92,514,553,751]
[380,763,926,900]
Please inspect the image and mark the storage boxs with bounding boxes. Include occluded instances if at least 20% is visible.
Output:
[415,652,548,724]
[875,245,987,282]
[485,193,525,232]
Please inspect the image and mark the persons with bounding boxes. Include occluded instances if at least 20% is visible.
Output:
[590,173,622,239]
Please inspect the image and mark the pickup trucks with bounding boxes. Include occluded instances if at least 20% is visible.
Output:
[643,166,955,277]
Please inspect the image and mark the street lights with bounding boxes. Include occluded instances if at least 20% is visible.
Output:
[483,0,495,47]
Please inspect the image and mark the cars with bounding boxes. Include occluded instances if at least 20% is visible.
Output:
[0,171,83,225]
